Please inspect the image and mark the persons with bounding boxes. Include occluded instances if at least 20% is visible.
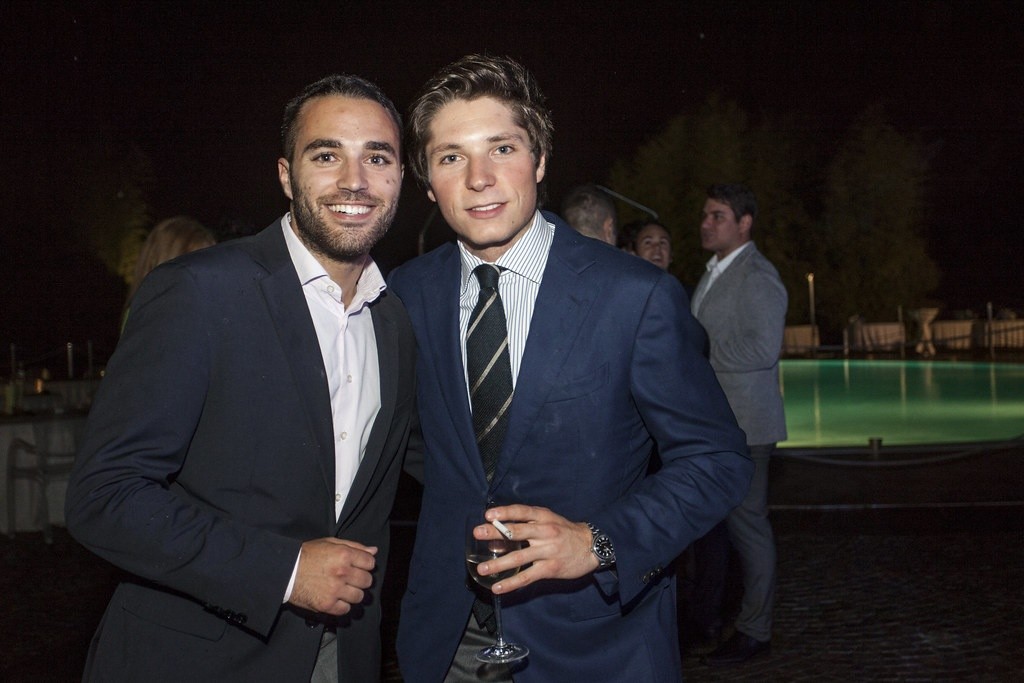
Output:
[621,220,673,270]
[691,182,789,664]
[121,216,218,339]
[64,72,426,682]
[560,188,618,247]
[388,53,757,682]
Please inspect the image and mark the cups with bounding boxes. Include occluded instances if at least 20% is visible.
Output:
[869,437,882,452]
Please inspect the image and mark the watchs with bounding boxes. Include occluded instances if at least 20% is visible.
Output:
[587,522,617,570]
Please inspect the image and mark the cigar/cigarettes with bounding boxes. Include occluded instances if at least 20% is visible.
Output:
[493,520,513,540]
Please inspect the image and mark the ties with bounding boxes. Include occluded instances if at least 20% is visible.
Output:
[465,262,517,634]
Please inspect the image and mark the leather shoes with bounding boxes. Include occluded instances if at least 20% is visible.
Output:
[700,629,771,668]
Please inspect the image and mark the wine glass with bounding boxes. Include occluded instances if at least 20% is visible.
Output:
[464,507,531,664]
